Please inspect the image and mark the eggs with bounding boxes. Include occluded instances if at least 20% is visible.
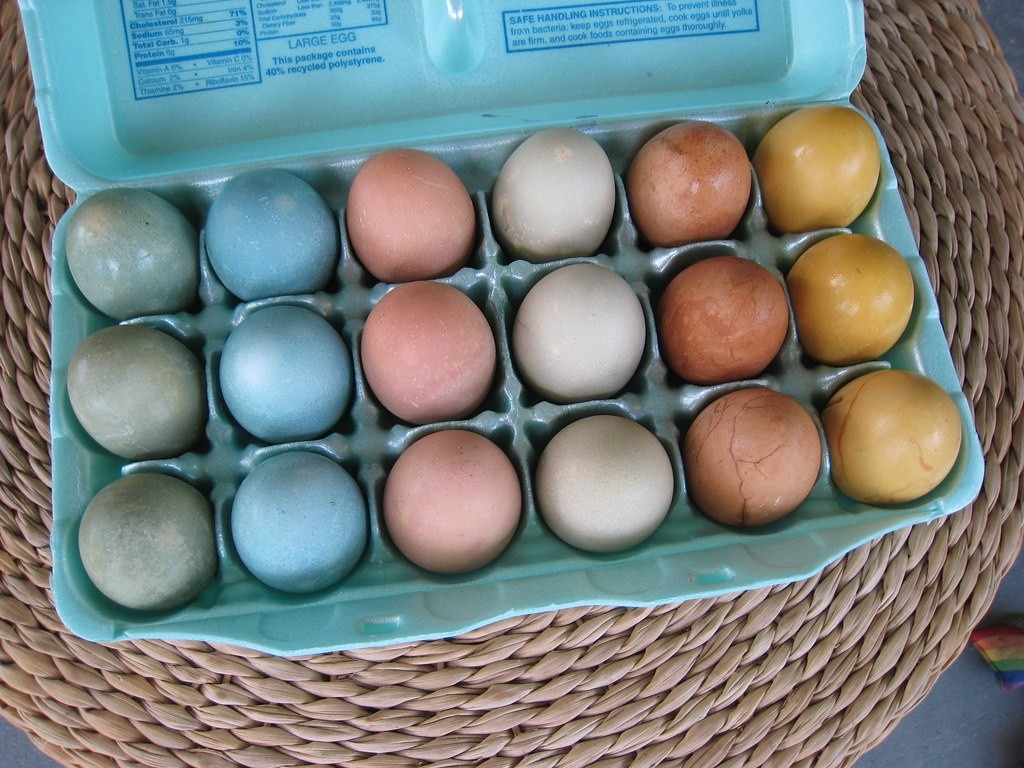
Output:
[64,104,963,616]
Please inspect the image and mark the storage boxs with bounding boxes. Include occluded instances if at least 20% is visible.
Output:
[17,2,983,652]
